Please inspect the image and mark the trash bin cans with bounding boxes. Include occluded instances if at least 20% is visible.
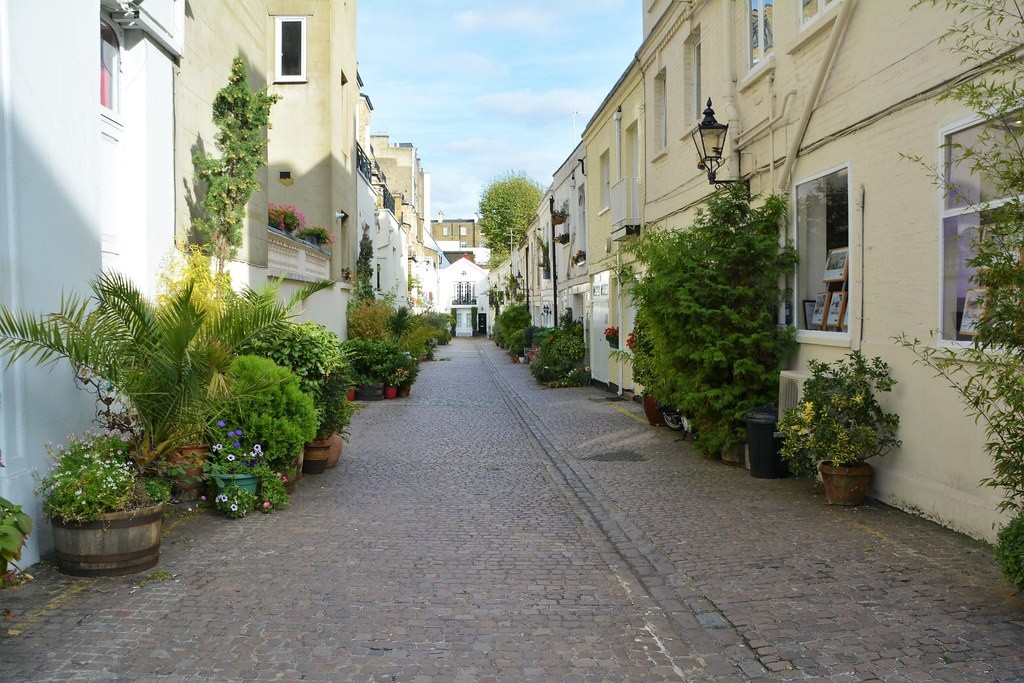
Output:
[742,404,796,480]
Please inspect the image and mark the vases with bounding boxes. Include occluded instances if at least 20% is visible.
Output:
[204,471,259,495]
[579,373,591,386]
[48,502,166,578]
[818,460,874,506]
[610,342,619,349]
[346,390,354,401]
[519,357,525,363]
[385,387,397,399]
[272,457,299,495]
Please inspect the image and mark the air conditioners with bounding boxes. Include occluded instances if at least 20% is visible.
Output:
[778,369,833,437]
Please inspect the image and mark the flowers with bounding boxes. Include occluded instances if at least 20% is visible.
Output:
[626,332,635,350]
[268,201,307,234]
[196,421,289,518]
[775,349,903,477]
[31,427,159,531]
[342,266,357,285]
[547,364,591,389]
[385,368,410,387]
[604,326,619,346]
[572,250,586,265]
[293,224,336,245]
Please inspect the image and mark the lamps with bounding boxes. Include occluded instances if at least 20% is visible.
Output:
[543,305,548,315]
[493,284,498,296]
[690,96,750,204]
[618,265,632,287]
[515,270,526,290]
[546,305,551,314]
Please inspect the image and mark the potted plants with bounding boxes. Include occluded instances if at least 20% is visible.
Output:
[434,328,452,346]
[631,309,667,426]
[604,206,722,461]
[166,400,209,490]
[551,208,569,225]
[552,233,569,245]
[302,347,373,475]
[341,338,416,401]
[673,182,800,468]
[326,400,366,468]
